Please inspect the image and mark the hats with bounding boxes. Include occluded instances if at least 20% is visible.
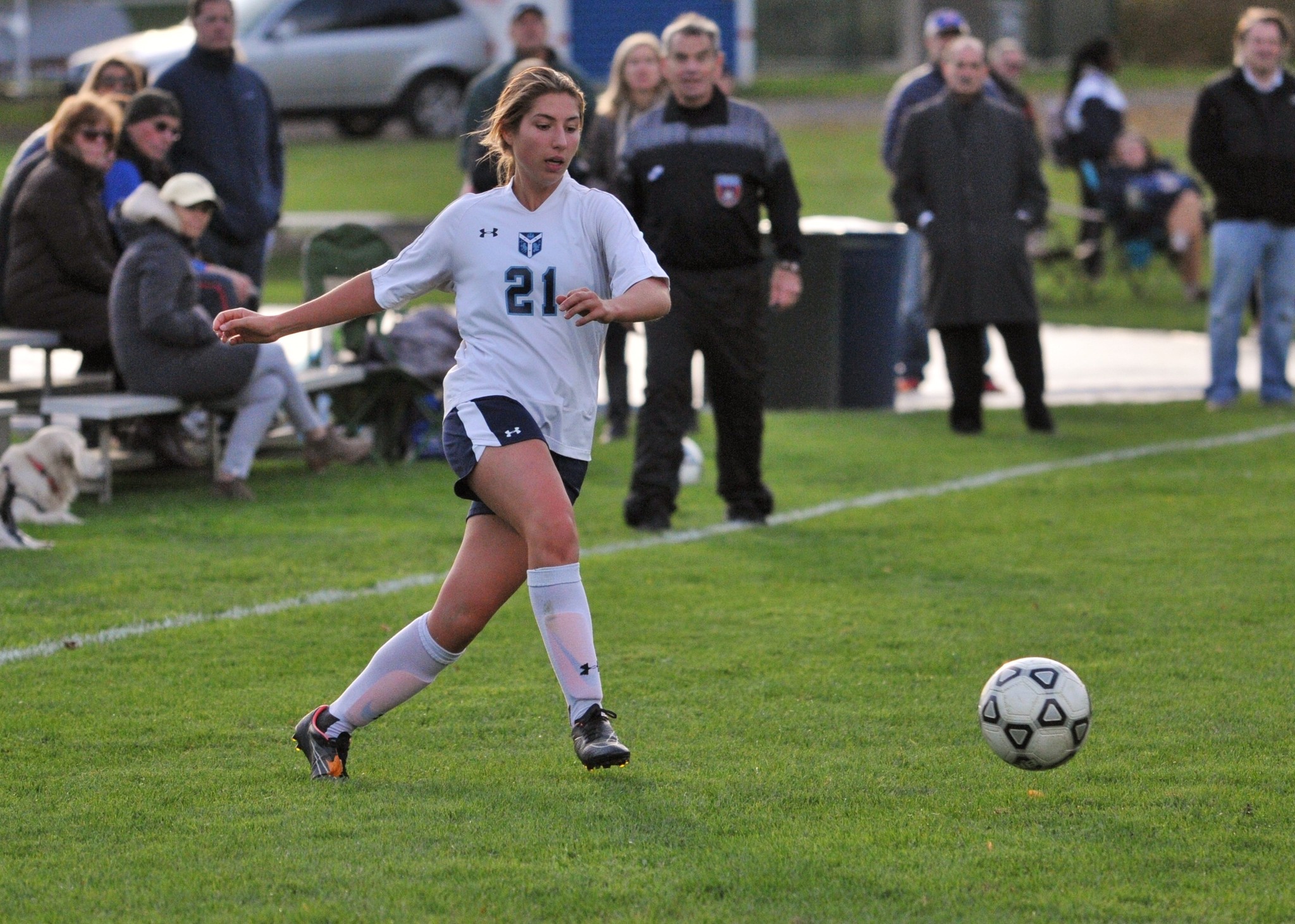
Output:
[157,172,221,210]
[924,5,970,39]
[512,3,544,21]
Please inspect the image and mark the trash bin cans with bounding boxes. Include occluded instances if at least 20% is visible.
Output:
[757,222,840,411]
[836,216,912,408]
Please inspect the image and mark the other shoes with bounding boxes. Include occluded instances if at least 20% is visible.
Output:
[893,376,919,394]
[1023,403,1053,431]
[978,379,1001,396]
[950,413,983,434]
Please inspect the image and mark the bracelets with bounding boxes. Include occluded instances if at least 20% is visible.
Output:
[774,259,800,273]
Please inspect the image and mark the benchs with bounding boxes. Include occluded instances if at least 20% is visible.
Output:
[0,324,390,511]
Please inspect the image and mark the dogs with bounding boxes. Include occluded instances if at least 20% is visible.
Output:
[0,424,106,551]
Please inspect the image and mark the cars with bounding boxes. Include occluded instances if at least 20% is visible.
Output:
[63,1,495,144]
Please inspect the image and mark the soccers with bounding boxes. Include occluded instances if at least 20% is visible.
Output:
[977,655,1092,774]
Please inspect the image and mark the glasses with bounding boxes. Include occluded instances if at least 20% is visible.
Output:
[146,118,182,135]
[99,75,134,87]
[74,127,114,142]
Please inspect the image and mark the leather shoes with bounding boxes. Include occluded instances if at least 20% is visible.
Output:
[208,467,256,502]
[303,428,374,476]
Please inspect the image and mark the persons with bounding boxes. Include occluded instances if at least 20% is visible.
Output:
[984,37,1045,160]
[1058,34,1126,275]
[612,12,803,533]
[889,34,1054,431]
[208,64,674,771]
[101,86,253,468]
[458,2,595,203]
[1104,129,1209,301]
[0,92,157,468]
[1184,5,1295,412]
[462,56,592,197]
[152,0,285,314]
[583,31,701,443]
[2,55,138,179]
[108,171,372,501]
[877,6,1006,391]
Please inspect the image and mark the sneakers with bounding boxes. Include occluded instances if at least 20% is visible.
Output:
[290,704,352,780]
[570,703,631,772]
